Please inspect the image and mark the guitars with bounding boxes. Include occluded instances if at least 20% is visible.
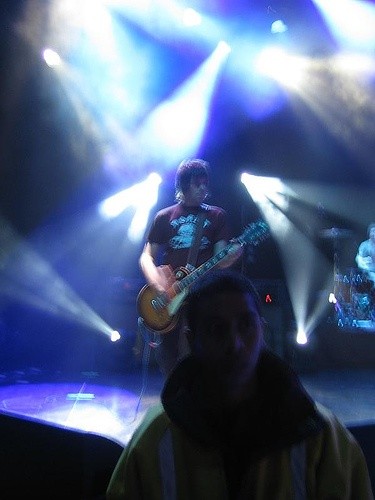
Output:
[135,220,269,334]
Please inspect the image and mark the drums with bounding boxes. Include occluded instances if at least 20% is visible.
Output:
[334,273,372,307]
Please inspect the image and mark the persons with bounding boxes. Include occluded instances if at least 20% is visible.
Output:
[105,271,375,500]
[355,224,375,278]
[138,158,242,380]
